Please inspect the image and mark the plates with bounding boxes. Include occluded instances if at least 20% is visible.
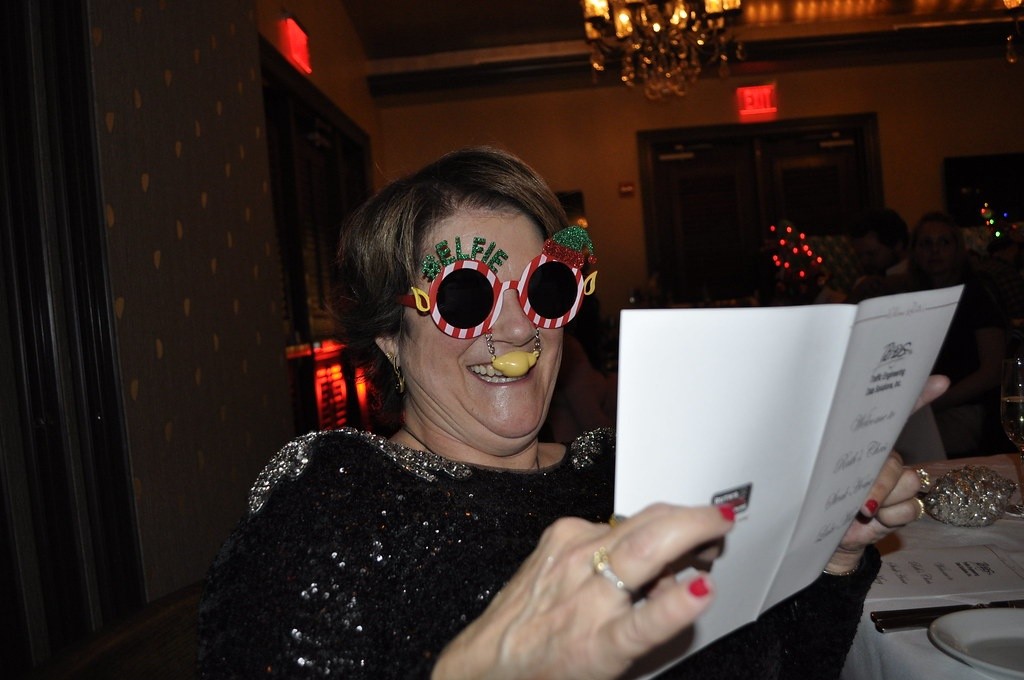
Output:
[931,607,1024,680]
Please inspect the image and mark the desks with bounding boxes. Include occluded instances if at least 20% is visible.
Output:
[839,452,1024,680]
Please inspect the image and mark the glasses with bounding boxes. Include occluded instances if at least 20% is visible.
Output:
[392,219,598,338]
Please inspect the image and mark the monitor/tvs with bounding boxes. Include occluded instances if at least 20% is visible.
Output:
[944,152,1024,227]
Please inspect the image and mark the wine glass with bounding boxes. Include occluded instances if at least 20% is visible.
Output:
[1000,360,1024,517]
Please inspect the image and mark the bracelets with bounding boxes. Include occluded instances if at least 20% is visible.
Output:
[821,562,864,577]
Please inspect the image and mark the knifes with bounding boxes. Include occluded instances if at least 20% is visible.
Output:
[871,599,1024,623]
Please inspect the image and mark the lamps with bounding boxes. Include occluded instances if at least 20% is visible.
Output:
[581,0,744,106]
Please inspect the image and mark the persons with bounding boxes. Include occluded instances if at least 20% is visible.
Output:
[195,146,928,680]
[549,294,617,446]
[639,189,1024,464]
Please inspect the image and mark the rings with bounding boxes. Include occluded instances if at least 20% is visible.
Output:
[594,545,630,590]
[913,496,926,520]
[900,463,931,492]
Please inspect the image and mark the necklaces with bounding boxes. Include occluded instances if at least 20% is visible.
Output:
[403,424,540,470]
